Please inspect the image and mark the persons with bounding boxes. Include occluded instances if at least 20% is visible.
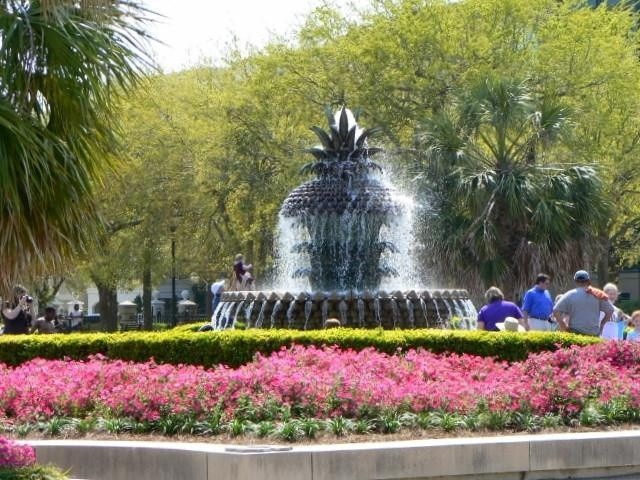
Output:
[474,268,640,345]
[233,271,258,291]
[231,252,258,284]
[0,283,37,336]
[210,270,239,326]
[31,304,84,334]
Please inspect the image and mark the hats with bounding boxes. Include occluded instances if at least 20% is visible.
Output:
[574,270,589,281]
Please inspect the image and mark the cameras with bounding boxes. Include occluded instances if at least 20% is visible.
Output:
[20,295,32,303]
